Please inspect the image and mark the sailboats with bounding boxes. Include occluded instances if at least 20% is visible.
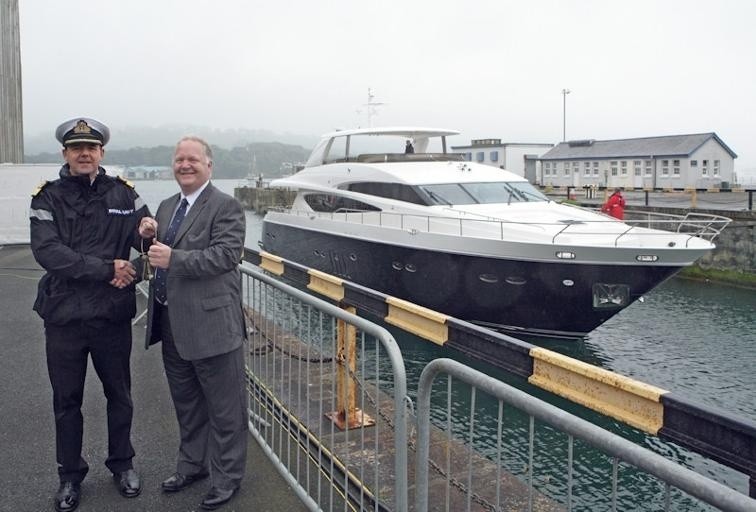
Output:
[244,152,256,180]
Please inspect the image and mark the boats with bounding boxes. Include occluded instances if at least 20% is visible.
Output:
[257,87,733,341]
[123,165,172,180]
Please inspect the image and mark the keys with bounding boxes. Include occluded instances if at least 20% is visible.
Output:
[139,255,154,280]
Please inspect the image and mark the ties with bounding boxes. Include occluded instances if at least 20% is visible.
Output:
[154,199,189,304]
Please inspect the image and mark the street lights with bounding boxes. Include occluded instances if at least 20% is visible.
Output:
[561,88,571,142]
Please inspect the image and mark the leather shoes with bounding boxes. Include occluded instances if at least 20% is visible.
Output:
[162,472,210,492]
[54,481,81,511]
[198,486,239,509]
[113,470,142,498]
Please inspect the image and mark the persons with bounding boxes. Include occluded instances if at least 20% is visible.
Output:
[405,139,414,154]
[27,118,157,511]
[108,135,249,511]
[601,187,627,222]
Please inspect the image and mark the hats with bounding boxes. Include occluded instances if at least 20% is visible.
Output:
[55,116,112,148]
